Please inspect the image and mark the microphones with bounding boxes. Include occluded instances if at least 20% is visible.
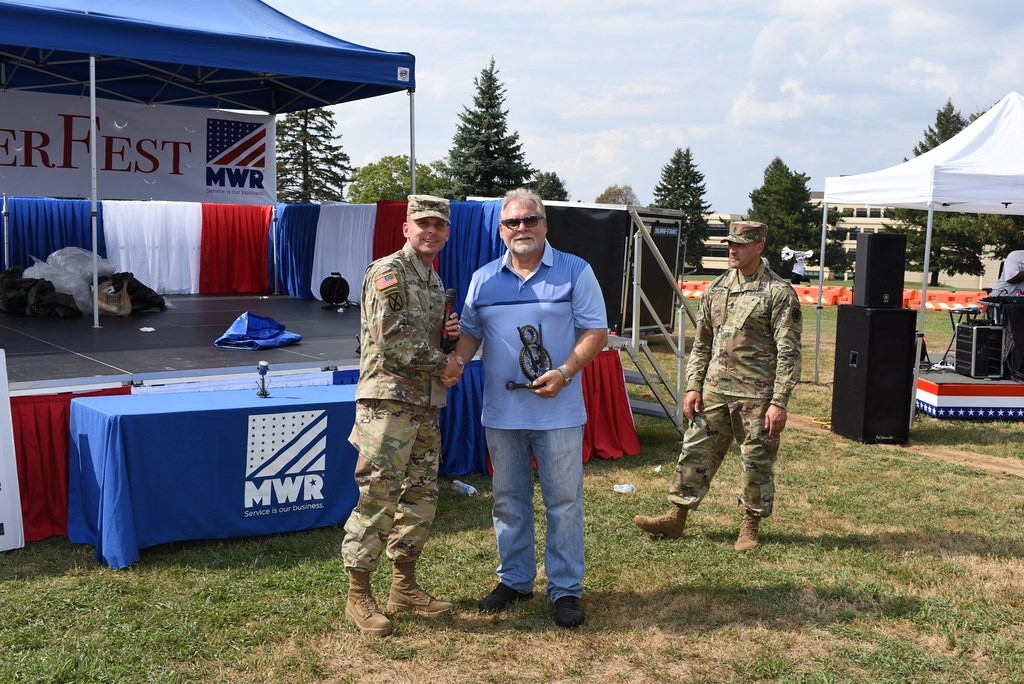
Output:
[440,289,457,350]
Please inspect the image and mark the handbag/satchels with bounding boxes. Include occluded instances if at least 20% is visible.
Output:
[88,272,132,316]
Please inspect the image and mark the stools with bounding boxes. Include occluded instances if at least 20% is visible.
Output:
[939,309,982,372]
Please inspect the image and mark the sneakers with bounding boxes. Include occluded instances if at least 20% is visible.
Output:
[482,583,534,612]
[555,596,584,629]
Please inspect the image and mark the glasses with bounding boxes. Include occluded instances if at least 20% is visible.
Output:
[500,215,544,230]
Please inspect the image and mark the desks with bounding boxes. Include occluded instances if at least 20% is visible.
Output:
[979,300,1023,378]
[68,383,358,570]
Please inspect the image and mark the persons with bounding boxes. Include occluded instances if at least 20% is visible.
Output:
[341,194,464,634]
[634,221,803,551]
[978,231,1024,311]
[438,186,608,628]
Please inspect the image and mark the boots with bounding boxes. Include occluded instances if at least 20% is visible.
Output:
[344,572,392,635]
[388,561,455,618]
[735,514,761,552]
[634,503,689,539]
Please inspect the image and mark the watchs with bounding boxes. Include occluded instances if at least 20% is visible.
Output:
[559,366,572,386]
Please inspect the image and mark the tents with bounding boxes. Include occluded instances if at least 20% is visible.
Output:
[815,91,1024,427]
[0,0,416,329]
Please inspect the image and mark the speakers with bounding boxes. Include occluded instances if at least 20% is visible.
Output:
[854,233,907,308]
[829,303,917,446]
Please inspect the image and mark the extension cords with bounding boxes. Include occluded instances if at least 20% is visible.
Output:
[931,364,955,370]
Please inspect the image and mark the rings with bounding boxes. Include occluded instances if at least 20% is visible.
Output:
[458,325,461,330]
[779,427,782,428]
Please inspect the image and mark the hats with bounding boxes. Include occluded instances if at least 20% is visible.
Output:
[720,221,768,244]
[407,194,451,224]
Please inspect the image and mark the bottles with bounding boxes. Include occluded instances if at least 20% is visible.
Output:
[450,479,477,497]
[613,484,637,493]
